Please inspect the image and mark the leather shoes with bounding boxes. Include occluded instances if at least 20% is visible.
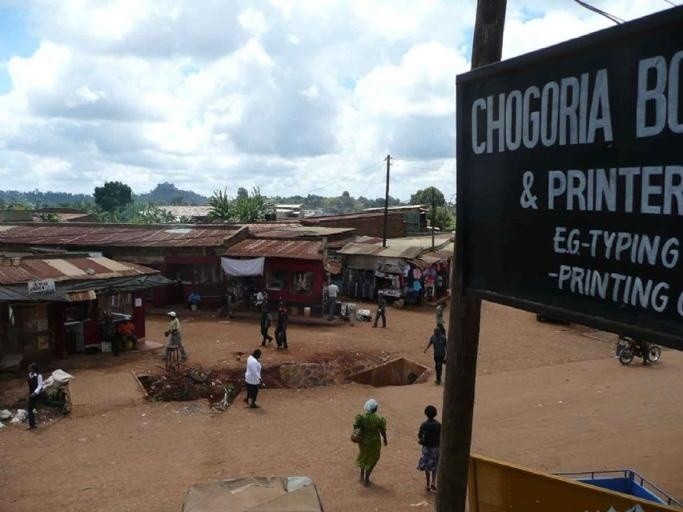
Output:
[425,485,430,491]
[431,485,436,490]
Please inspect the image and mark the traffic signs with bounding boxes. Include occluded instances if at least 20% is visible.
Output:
[456,4,682,348]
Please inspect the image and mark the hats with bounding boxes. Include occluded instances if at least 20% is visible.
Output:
[364,399,377,411]
[168,311,176,316]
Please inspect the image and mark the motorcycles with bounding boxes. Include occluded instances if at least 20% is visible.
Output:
[618,336,661,365]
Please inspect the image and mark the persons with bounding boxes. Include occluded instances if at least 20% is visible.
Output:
[254,288,268,313]
[23,363,65,429]
[188,289,202,312]
[243,349,265,408]
[258,304,273,347]
[326,281,341,322]
[370,291,386,329]
[435,302,446,340]
[353,399,388,487]
[631,338,651,366]
[423,326,447,386]
[163,311,187,362]
[275,301,287,350]
[436,270,443,300]
[415,405,443,491]
[118,317,138,351]
[101,321,122,357]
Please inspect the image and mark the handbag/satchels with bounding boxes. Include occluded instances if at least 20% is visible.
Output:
[351,428,361,442]
